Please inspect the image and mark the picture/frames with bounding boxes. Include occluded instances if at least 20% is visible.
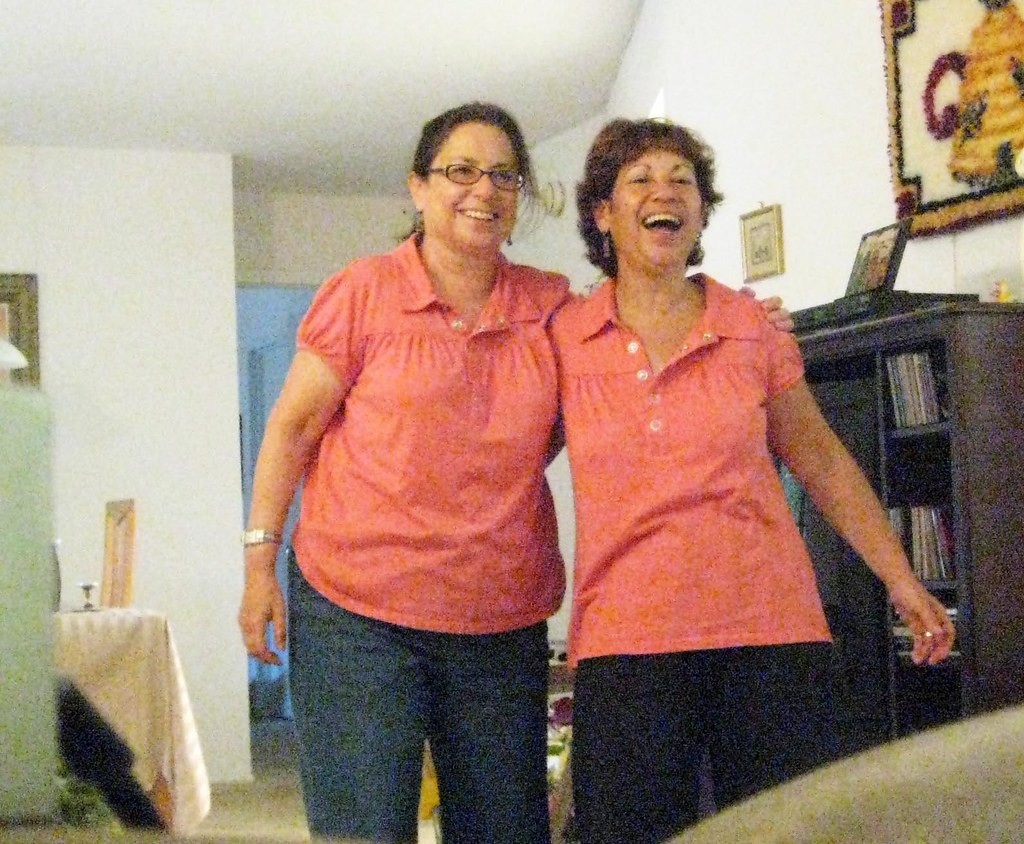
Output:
[739,204,785,283]
[882,0,1024,236]
[844,218,914,297]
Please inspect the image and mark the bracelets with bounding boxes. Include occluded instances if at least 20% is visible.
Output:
[245,529,284,545]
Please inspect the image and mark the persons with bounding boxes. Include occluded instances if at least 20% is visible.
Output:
[540,115,954,844]
[243,103,796,844]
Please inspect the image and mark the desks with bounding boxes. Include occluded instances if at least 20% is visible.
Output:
[53,608,211,835]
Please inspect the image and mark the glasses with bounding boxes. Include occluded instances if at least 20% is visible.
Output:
[424,164,526,192]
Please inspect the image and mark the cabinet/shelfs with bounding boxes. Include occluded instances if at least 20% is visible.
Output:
[771,302,1024,776]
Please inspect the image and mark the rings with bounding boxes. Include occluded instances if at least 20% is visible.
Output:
[923,632,933,637]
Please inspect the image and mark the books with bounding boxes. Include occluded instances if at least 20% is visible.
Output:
[893,607,961,661]
[884,349,942,428]
[887,499,951,582]
